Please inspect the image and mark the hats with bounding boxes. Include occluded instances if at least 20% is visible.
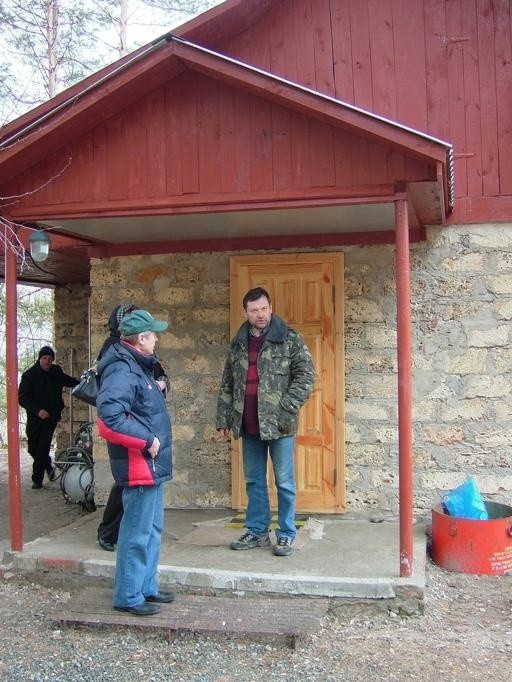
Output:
[118,309,168,336]
[39,346,54,359]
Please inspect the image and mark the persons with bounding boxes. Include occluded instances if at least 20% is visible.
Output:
[94,309,176,618]
[214,285,317,558]
[17,346,81,489]
[93,303,170,551]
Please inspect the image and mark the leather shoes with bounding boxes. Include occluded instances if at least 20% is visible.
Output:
[99,534,115,550]
[114,601,161,614]
[145,590,175,602]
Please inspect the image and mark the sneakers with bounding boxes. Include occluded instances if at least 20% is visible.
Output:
[274,535,295,556]
[48,468,55,479]
[32,482,42,488]
[232,530,271,549]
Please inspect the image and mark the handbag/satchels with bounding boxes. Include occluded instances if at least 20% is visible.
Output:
[72,340,109,407]
[442,478,489,520]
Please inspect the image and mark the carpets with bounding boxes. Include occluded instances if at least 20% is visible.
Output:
[224,512,311,531]
[173,524,309,550]
[191,517,325,542]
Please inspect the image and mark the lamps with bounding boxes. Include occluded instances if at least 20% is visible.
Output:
[28,225,51,263]
